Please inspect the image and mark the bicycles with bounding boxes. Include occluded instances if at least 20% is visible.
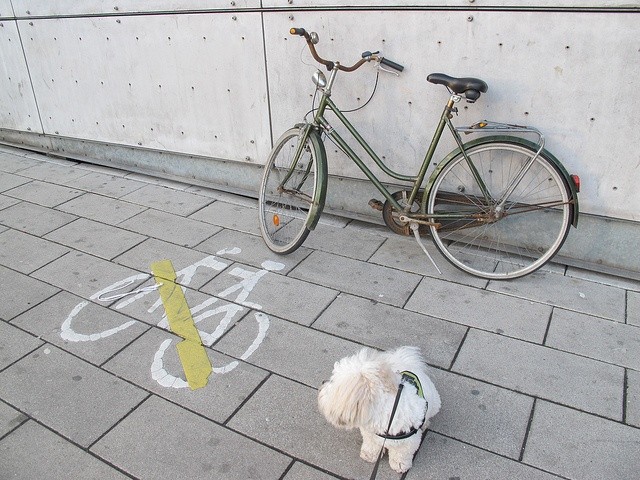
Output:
[258,28,580,280]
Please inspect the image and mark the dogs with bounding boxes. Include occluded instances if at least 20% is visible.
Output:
[317,346,442,473]
